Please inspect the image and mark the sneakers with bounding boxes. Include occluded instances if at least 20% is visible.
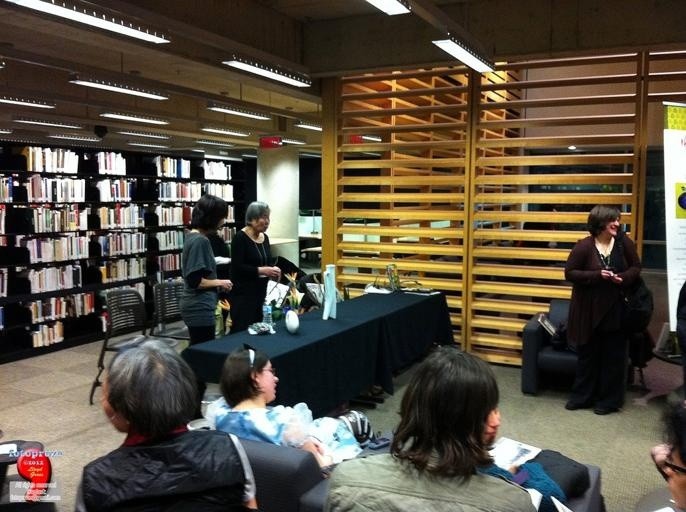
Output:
[565,400,619,414]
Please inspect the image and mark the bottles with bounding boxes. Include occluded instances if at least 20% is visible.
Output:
[263,296,272,326]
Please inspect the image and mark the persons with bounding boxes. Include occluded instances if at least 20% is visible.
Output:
[231,202,281,334]
[649,396,686,512]
[208,344,380,468]
[207,208,231,329]
[322,345,536,512]
[565,205,642,416]
[483,406,500,445]
[181,194,234,347]
[73,336,260,512]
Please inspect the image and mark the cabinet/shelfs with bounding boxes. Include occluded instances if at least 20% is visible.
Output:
[152,150,202,322]
[91,148,151,342]
[0,144,91,364]
[203,160,246,258]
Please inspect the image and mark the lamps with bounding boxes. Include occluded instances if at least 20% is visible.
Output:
[0,0,172,45]
[66,51,168,100]
[430,36,495,72]
[220,53,311,88]
[366,0,410,16]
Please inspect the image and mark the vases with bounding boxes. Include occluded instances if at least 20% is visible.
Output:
[284,308,300,335]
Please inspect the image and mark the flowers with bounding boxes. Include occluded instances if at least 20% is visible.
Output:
[284,272,300,310]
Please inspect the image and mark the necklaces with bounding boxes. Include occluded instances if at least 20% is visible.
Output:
[598,245,613,270]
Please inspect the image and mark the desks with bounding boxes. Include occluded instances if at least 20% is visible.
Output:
[179,292,453,418]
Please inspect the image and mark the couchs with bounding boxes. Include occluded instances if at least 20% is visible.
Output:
[237,438,601,512]
[522,300,577,394]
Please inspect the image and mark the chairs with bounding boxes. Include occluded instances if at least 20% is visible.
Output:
[151,282,190,340]
[88,287,172,404]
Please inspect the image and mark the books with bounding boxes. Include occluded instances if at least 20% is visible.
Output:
[199,160,236,250]
[1,146,95,347]
[538,312,558,337]
[485,437,541,472]
[153,156,201,286]
[84,152,147,333]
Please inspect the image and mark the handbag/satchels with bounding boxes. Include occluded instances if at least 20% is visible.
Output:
[516,450,590,498]
[622,285,654,333]
